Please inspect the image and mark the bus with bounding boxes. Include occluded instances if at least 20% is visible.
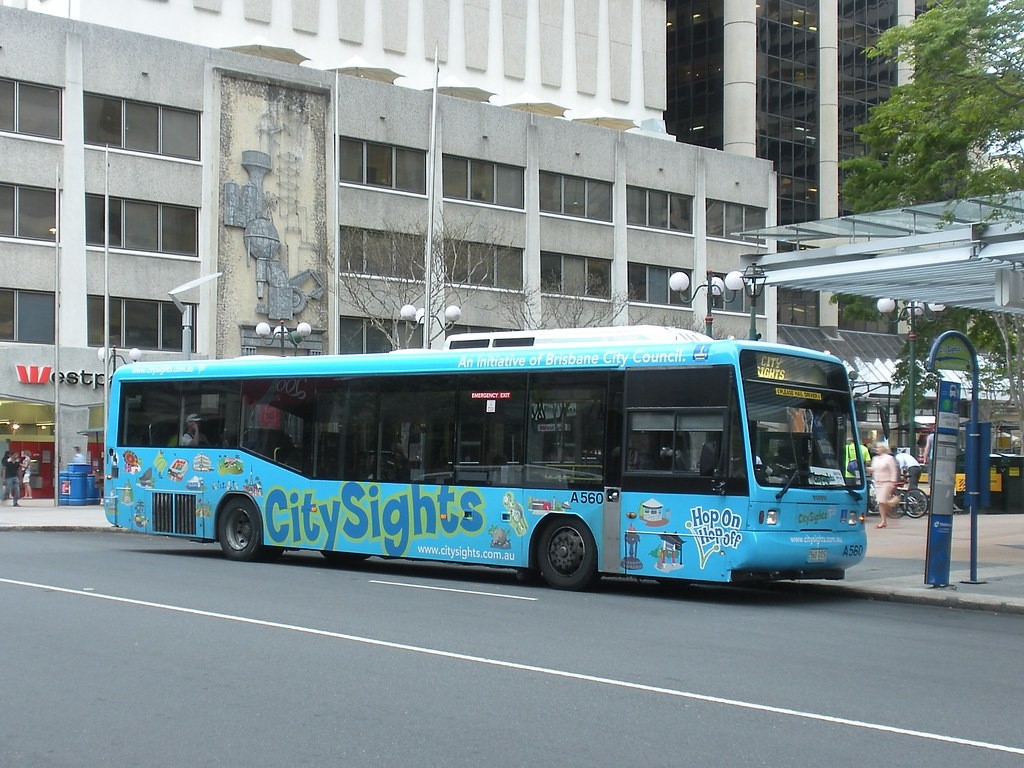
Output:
[103,324,868,587]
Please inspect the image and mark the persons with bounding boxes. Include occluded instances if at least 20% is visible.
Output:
[1,451,15,500]
[924,425,937,487]
[19,450,33,500]
[845,435,871,486]
[1,451,28,507]
[865,441,898,529]
[181,413,211,447]
[894,453,922,499]
[69,446,86,464]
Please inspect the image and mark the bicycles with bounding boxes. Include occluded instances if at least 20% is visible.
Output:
[864,471,908,519]
[894,481,929,517]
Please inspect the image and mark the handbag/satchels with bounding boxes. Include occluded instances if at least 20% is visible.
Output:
[847,460,866,478]
[886,488,901,509]
[19,468,27,481]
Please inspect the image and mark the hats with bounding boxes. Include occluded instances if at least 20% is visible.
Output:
[185,414,202,423]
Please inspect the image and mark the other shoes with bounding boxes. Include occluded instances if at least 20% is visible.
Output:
[14,503,21,508]
[876,522,887,529]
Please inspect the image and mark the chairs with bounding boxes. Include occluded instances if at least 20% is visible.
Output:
[700,440,719,476]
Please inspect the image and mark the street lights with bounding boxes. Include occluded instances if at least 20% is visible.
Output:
[400,304,462,350]
[739,260,768,341]
[97,343,142,387]
[169,273,223,360]
[668,270,746,340]
[876,297,945,454]
[254,320,312,357]
